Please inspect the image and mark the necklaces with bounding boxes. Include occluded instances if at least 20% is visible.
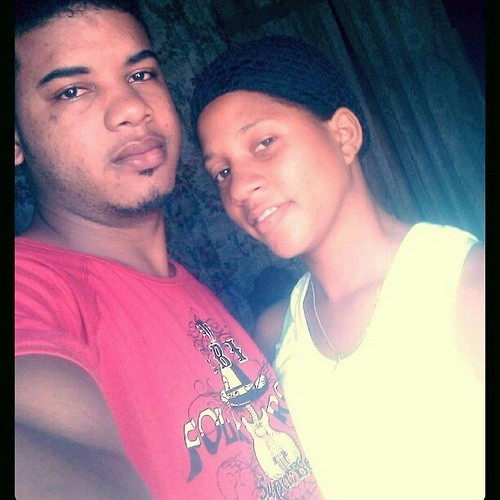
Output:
[307,280,384,357]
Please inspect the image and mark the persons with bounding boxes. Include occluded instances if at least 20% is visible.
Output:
[193,37,486,499]
[14,0,325,500]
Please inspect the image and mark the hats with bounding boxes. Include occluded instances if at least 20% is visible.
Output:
[190,35,371,163]
